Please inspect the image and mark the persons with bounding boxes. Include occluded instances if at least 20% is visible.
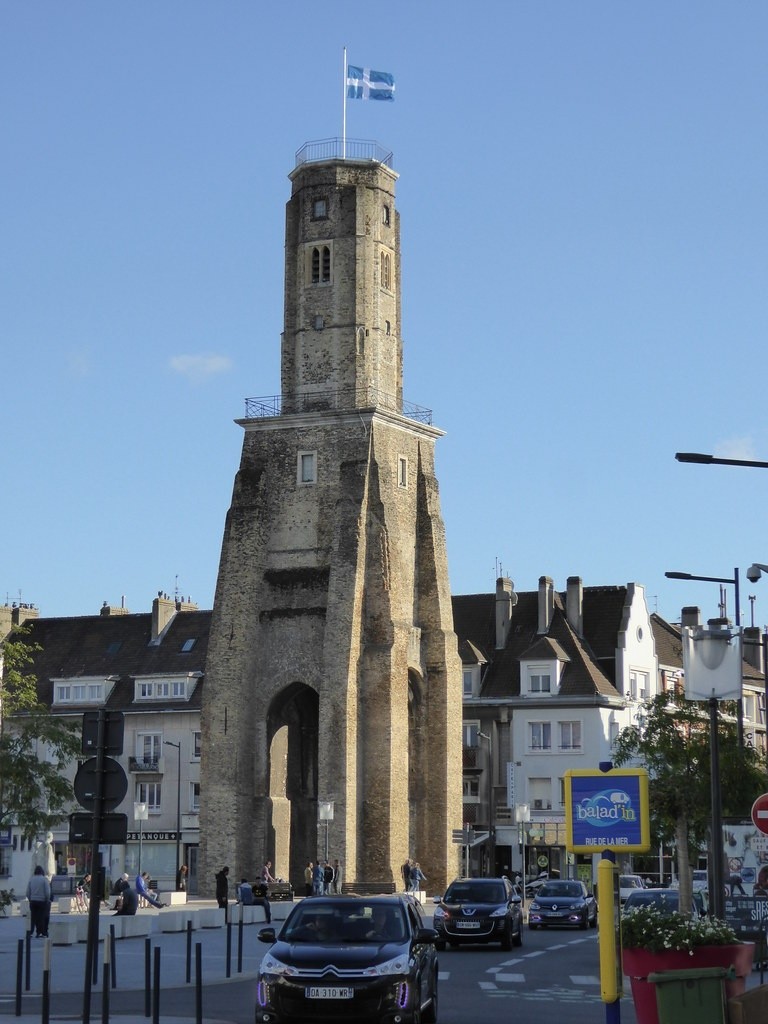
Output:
[112,882,138,916]
[136,872,169,909]
[179,865,188,891]
[304,859,344,896]
[113,873,130,894]
[215,866,229,924]
[25,865,53,938]
[79,873,111,912]
[401,859,427,891]
[261,861,275,883]
[233,877,272,924]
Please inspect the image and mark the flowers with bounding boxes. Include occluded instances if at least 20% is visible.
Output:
[614,902,738,957]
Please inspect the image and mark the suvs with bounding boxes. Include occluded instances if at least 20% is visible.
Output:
[431,876,525,950]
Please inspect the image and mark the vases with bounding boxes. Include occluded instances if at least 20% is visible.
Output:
[623,942,754,977]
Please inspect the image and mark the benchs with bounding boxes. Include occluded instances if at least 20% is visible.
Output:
[234,882,295,902]
[341,882,396,895]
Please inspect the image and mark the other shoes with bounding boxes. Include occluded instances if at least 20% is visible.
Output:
[31,932,47,938]
[268,917,271,924]
[158,904,164,909]
[147,905,155,908]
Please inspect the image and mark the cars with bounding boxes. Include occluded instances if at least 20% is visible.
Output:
[528,880,598,930]
[619,874,650,905]
[691,869,708,893]
[254,894,440,1024]
[622,888,698,919]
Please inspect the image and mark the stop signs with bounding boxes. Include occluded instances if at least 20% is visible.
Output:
[751,792,768,834]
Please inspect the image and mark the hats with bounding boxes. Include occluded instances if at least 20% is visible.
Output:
[324,860,328,863]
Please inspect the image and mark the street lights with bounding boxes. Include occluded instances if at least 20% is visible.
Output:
[666,567,744,747]
[133,801,149,875]
[163,740,181,891]
[680,624,742,919]
[319,801,335,862]
[513,803,531,908]
